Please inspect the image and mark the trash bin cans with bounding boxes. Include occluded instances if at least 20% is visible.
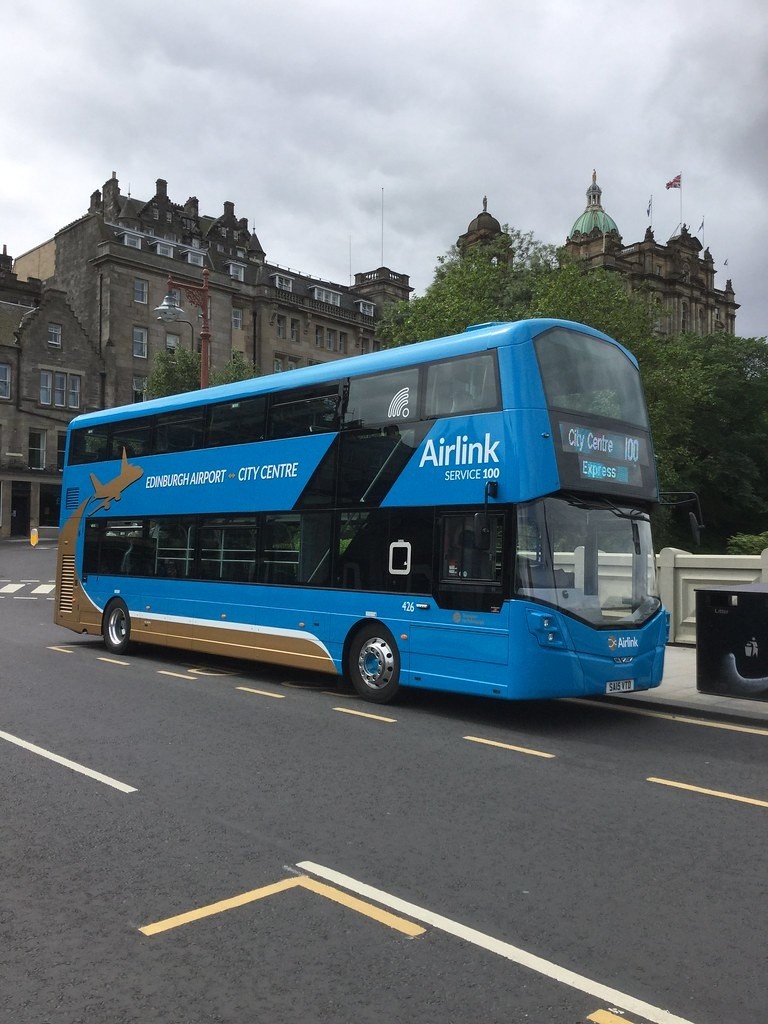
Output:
[693,581,768,705]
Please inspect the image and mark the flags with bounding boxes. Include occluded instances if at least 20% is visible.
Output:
[665,175,680,189]
[647,199,651,216]
[698,222,704,232]
[724,260,727,265]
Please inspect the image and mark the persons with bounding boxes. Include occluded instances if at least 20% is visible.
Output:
[680,222,691,238]
[704,246,714,261]
[645,226,654,241]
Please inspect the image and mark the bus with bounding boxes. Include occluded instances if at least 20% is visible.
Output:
[54,318,705,704]
